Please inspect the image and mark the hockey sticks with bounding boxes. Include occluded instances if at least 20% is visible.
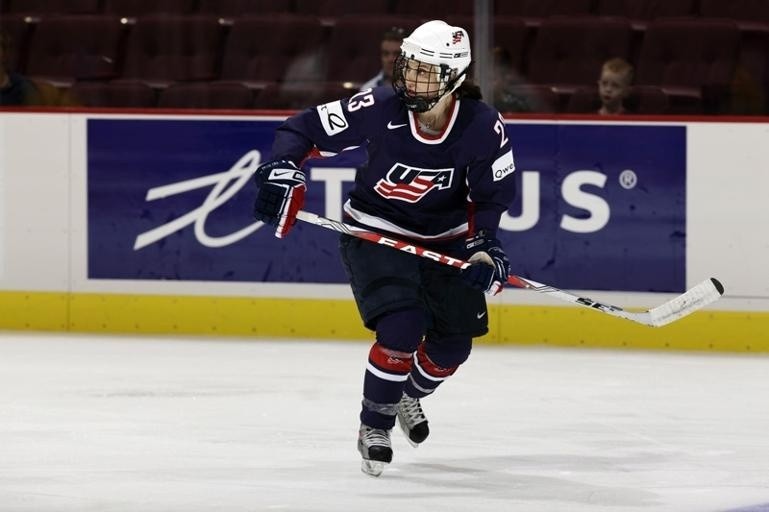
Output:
[297,211,724,326]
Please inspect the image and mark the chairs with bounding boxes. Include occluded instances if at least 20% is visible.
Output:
[1,0,768,118]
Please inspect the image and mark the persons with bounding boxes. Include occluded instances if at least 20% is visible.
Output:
[592,57,639,115]
[251,17,518,464]
[355,26,408,93]
[490,42,531,112]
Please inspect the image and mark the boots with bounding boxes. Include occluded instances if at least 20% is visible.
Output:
[397,390,429,443]
[357,422,393,463]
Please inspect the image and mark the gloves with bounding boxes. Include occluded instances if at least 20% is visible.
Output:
[253,159,307,239]
[462,237,512,296]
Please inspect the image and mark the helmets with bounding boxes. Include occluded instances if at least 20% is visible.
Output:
[400,19,472,87]
